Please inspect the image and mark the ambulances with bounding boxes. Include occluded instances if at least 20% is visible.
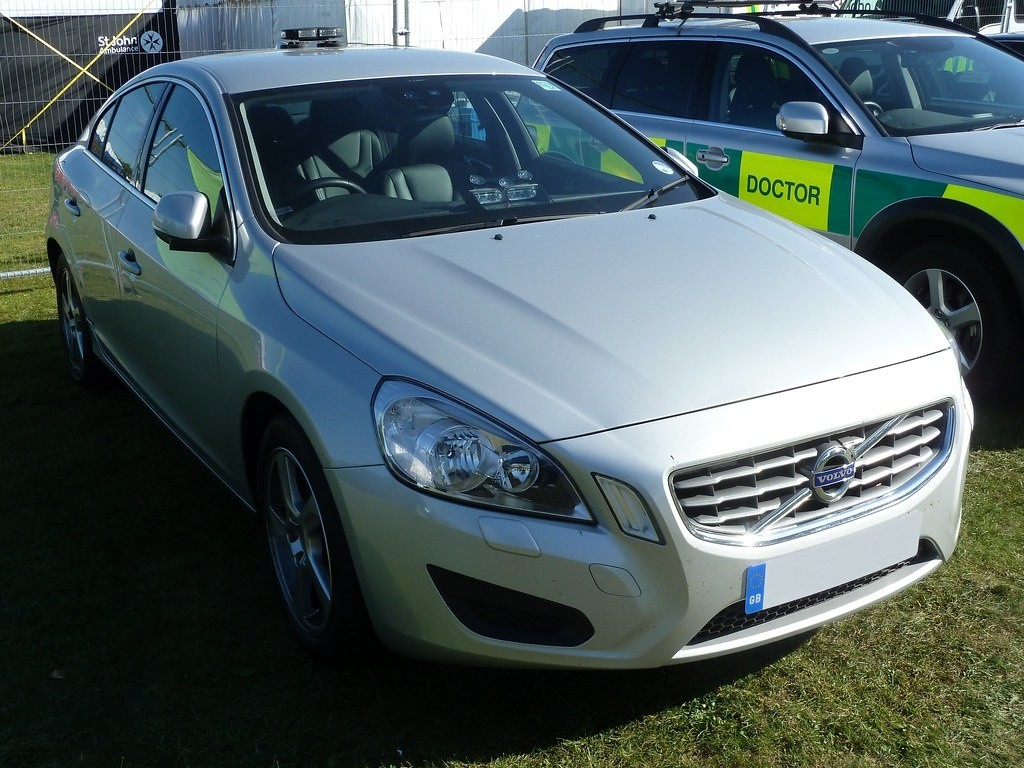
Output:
[516,0,1024,406]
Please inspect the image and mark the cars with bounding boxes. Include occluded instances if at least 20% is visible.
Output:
[44,26,975,675]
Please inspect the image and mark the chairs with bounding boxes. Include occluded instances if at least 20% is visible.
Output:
[245,107,465,208]
[625,51,878,137]
